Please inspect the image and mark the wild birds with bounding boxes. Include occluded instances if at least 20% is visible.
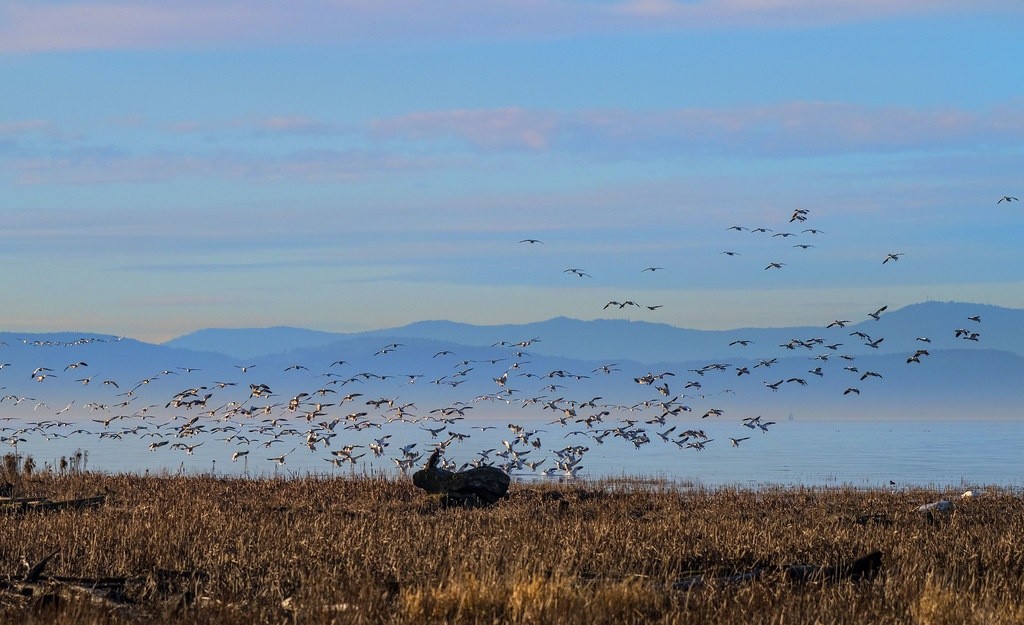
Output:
[772,233,797,237]
[801,229,824,234]
[868,305,888,321]
[907,349,930,364]
[883,253,905,264]
[793,245,814,249]
[720,252,741,256]
[563,268,591,277]
[519,239,544,244]
[955,329,980,342]
[0,319,887,477]
[603,301,663,310]
[968,316,980,323]
[765,262,787,270]
[916,337,932,344]
[789,209,810,223]
[752,228,773,233]
[998,196,1019,204]
[727,226,749,231]
[642,268,662,271]
